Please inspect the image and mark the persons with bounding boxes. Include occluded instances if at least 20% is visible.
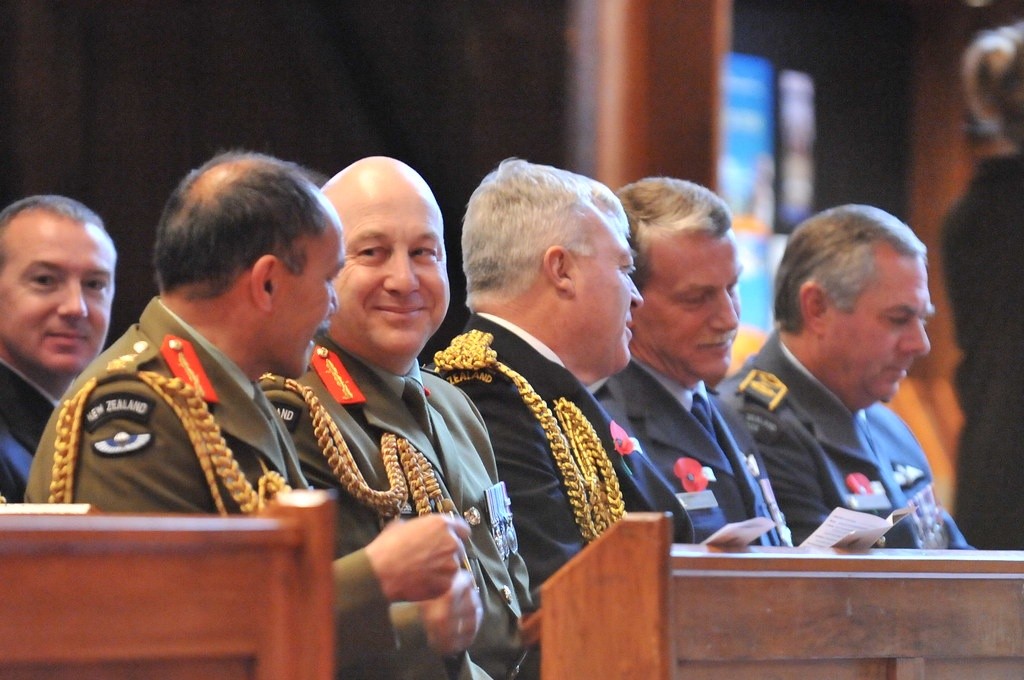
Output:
[0,196,116,503]
[427,162,694,680]
[258,158,537,680]
[27,152,481,680]
[595,177,793,547]
[723,204,970,548]
[943,20,1023,552]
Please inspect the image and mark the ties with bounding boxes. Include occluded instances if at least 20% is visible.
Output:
[691,394,716,444]
[403,378,436,449]
[251,381,279,444]
